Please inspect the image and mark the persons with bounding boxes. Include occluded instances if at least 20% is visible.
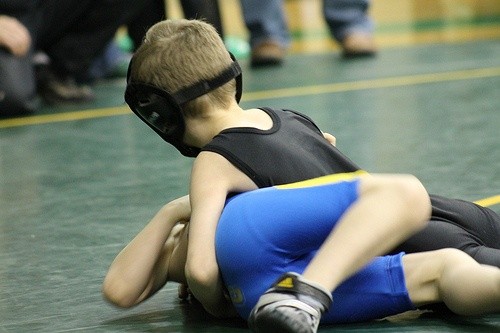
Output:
[99,16,500,332]
[0,0,375,114]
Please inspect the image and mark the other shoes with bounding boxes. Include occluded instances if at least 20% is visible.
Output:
[251,41,285,66]
[45,83,93,105]
[343,34,377,56]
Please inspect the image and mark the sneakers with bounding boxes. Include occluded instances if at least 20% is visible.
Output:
[247,270,333,333]
